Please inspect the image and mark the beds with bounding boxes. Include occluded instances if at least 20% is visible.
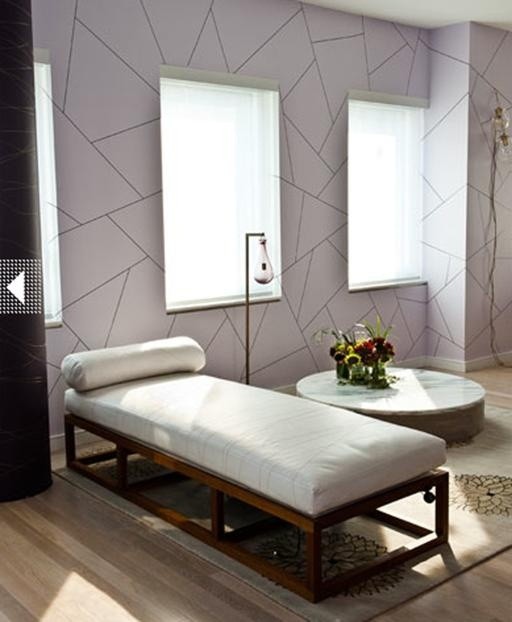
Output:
[63,371,449,604]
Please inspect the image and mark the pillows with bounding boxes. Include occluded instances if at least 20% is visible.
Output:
[61,336,205,391]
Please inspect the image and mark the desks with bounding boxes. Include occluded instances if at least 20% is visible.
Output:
[295,367,485,445]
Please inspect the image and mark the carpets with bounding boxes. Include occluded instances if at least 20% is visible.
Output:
[52,404,511,622]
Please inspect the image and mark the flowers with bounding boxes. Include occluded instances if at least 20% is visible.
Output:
[311,298,351,386]
[344,330,369,386]
[353,314,401,390]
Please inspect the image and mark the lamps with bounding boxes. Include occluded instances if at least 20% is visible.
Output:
[491,106,511,155]
[245,232,274,383]
